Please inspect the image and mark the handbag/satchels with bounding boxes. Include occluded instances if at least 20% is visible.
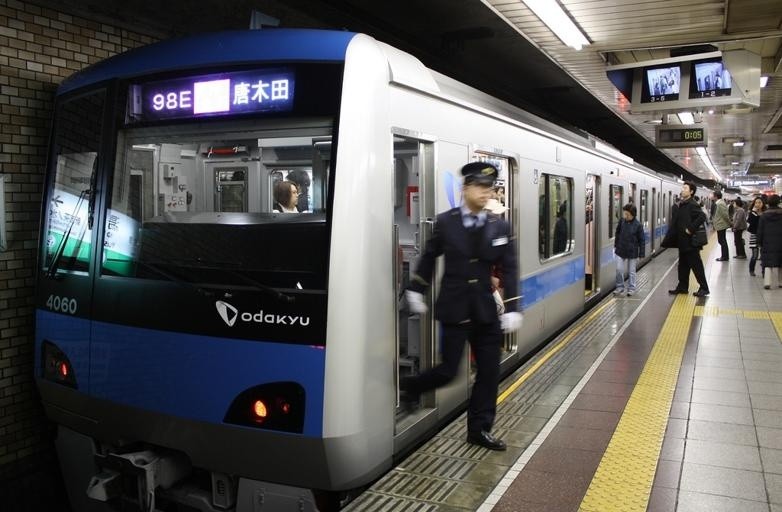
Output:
[687,223,708,247]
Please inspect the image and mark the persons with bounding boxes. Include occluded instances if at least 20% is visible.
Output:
[613,203,644,294]
[274,182,298,214]
[660,181,782,296]
[705,70,722,90]
[653,76,678,95]
[406,162,518,451]
[288,170,310,213]
[495,185,593,254]
[227,171,244,212]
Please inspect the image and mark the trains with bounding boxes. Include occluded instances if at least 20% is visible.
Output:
[26,24,710,511]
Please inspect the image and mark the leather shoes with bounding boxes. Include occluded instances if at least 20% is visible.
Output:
[402,377,420,412]
[466,431,507,451]
[669,288,689,294]
[693,288,710,296]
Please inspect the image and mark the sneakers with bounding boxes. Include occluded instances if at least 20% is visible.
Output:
[613,288,623,295]
[627,289,636,296]
[716,256,782,289]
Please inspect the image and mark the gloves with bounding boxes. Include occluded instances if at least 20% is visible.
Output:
[501,312,524,334]
[405,289,428,313]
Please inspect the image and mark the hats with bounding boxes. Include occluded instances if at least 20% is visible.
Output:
[461,162,499,185]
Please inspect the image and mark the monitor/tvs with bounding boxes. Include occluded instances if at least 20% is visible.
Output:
[688,56,732,100]
[640,63,681,104]
